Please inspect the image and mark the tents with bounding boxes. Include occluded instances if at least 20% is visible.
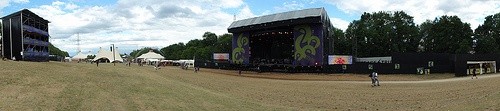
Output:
[136,51,165,65]
[71,51,96,61]
[92,47,124,63]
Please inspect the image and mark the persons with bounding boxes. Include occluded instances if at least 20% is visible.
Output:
[374,71,380,86]
[371,69,376,87]
[472,65,477,79]
[484,65,487,74]
